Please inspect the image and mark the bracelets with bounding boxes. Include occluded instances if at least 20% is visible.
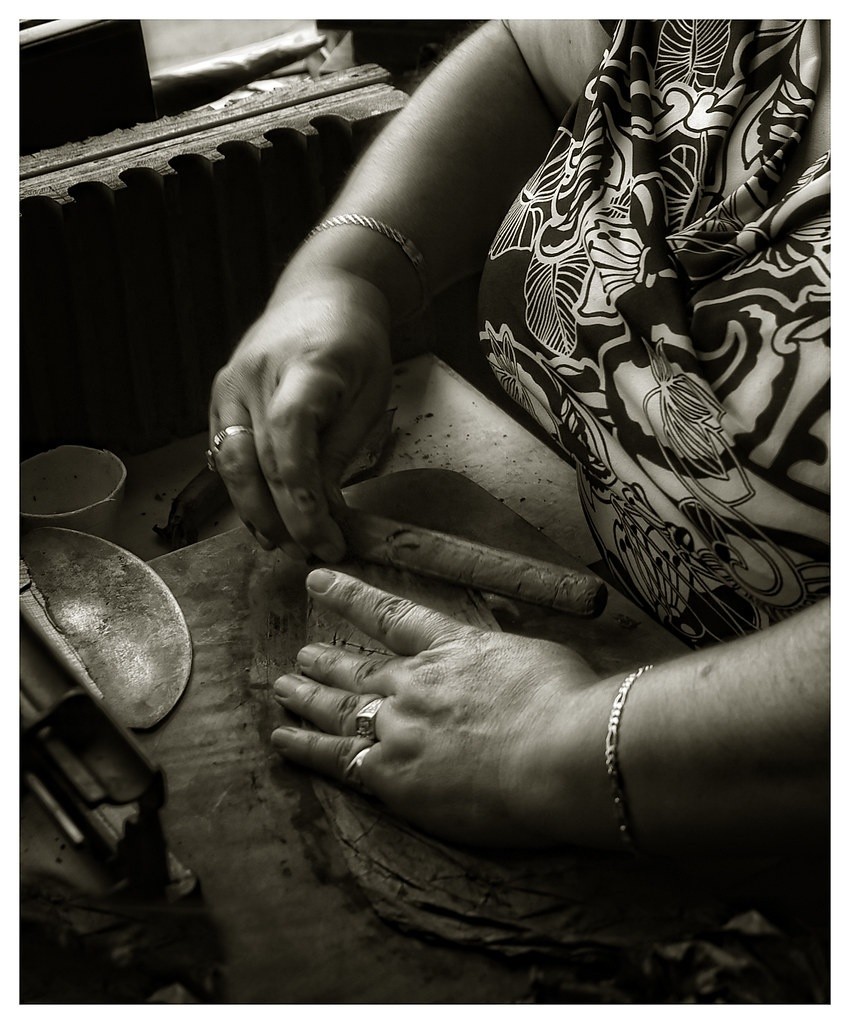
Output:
[303,214,431,326]
[604,664,654,854]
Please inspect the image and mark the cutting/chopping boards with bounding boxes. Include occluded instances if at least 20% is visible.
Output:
[123,464,830,1004]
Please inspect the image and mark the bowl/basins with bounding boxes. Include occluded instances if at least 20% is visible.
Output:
[20,444,128,538]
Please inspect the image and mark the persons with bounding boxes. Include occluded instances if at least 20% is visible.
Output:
[208,19,832,843]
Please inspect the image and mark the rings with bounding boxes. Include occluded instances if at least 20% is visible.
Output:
[204,425,254,473]
[356,699,384,740]
[342,746,373,788]
